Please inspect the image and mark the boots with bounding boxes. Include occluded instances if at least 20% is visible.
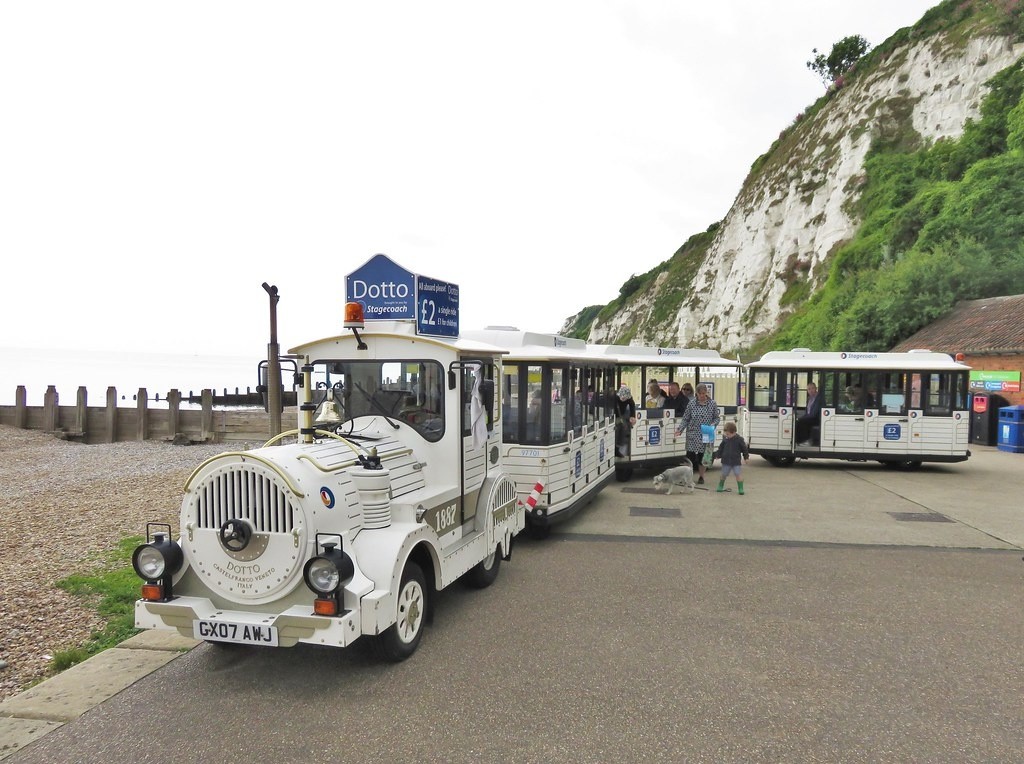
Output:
[615,447,625,458]
[717,478,724,491]
[738,481,744,494]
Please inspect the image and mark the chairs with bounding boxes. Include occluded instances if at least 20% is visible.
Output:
[369,394,404,418]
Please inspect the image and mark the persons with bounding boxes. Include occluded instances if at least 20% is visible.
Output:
[552,388,559,404]
[841,384,874,414]
[411,367,442,401]
[614,386,636,458]
[575,385,592,400]
[528,389,543,422]
[645,378,695,417]
[715,422,749,494]
[796,383,825,446]
[673,383,720,484]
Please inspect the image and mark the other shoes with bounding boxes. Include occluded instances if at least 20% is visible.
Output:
[698,476,704,484]
[800,440,813,446]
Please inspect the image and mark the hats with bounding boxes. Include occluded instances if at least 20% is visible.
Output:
[530,390,542,398]
[617,386,632,399]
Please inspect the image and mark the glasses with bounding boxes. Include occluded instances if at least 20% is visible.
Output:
[682,387,691,391]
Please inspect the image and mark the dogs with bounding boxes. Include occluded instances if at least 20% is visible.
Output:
[653,457,696,495]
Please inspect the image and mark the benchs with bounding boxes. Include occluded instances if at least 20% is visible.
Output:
[509,406,568,437]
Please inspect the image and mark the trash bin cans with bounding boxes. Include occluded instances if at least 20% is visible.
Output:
[954,389,975,445]
[997,404,1024,453]
[971,391,1002,446]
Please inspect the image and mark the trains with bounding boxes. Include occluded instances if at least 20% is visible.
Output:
[136,253,972,664]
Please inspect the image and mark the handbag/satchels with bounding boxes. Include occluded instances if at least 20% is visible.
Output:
[702,447,712,466]
[621,418,630,436]
